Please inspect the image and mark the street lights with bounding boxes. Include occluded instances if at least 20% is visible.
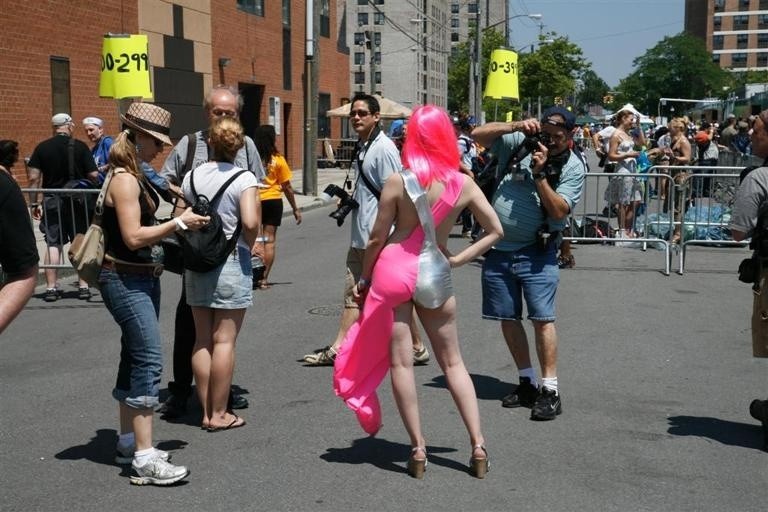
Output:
[468,10,540,122]
[511,34,558,120]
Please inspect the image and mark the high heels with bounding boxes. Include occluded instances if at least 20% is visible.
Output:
[408,447,428,479]
[470,446,490,478]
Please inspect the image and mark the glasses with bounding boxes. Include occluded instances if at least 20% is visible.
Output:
[350,111,368,116]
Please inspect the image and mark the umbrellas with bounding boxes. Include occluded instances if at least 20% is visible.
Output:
[328,94,412,121]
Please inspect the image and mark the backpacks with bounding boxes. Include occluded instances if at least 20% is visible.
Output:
[175,169,250,273]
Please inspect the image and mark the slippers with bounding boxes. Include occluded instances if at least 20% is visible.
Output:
[201,415,245,431]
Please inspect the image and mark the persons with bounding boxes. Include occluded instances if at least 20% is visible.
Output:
[397,108,756,269]
[0,137,19,170]
[173,119,259,429]
[28,112,302,302]
[299,94,429,365]
[472,107,585,419]
[349,105,504,480]
[158,86,270,417]
[727,108,768,431]
[95,103,212,487]
[2,170,39,336]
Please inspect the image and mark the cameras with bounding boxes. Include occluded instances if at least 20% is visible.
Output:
[192,199,211,216]
[323,183,359,227]
[523,129,543,153]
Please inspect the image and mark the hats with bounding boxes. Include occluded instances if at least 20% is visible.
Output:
[541,107,574,130]
[52,113,74,126]
[120,102,173,145]
[83,117,103,127]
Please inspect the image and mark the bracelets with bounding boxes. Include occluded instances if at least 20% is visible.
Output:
[361,276,371,286]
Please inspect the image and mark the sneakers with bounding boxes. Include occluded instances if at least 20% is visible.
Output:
[78,288,90,298]
[229,388,247,408]
[46,289,61,301]
[304,347,337,365]
[160,394,187,417]
[503,376,561,420]
[413,347,429,364]
[115,444,190,485]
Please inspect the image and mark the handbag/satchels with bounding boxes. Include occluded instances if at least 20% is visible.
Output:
[69,224,106,285]
